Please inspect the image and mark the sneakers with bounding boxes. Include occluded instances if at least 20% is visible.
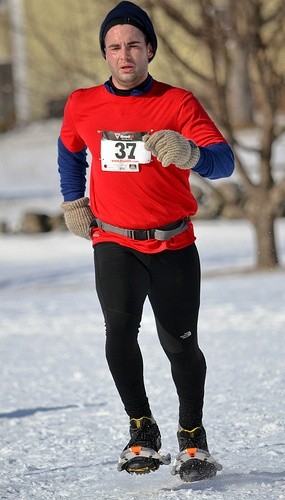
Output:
[117,417,171,475]
[171,424,222,483]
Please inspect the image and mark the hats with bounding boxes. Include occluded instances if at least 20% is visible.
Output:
[99,1,158,64]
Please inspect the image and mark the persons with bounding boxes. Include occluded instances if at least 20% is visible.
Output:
[59,0,234,477]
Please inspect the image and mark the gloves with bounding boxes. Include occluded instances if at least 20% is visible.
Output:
[142,130,200,169]
[61,196,96,240]
